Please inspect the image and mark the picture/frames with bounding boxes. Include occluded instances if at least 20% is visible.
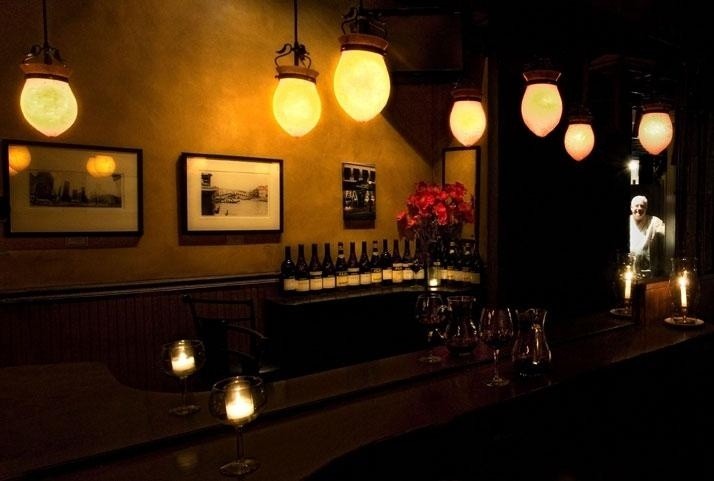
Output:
[179,151,285,236]
[1,139,145,238]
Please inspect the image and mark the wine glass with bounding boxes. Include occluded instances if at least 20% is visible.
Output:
[480,305,514,389]
[415,293,447,364]
[207,375,269,475]
[162,340,206,417]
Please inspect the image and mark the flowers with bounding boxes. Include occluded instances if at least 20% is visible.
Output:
[396,178,477,242]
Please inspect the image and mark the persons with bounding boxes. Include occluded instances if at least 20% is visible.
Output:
[629,195,666,272]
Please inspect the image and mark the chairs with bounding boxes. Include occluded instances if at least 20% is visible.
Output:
[179,294,280,389]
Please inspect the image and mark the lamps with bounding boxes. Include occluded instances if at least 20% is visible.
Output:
[333,0,391,124]
[444,57,490,148]
[632,94,676,157]
[272,1,322,138]
[518,54,567,145]
[559,102,599,171]
[18,1,79,138]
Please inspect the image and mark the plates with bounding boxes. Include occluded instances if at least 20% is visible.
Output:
[610,307,633,318]
[665,317,705,328]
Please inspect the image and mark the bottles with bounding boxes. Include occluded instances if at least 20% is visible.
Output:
[445,296,478,354]
[281,233,481,292]
[512,310,554,378]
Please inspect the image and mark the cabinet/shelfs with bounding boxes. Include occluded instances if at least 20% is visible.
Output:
[264,279,483,379]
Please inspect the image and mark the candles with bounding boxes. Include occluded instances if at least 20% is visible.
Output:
[225,396,254,420]
[623,274,631,299]
[173,356,195,374]
[680,283,687,308]
